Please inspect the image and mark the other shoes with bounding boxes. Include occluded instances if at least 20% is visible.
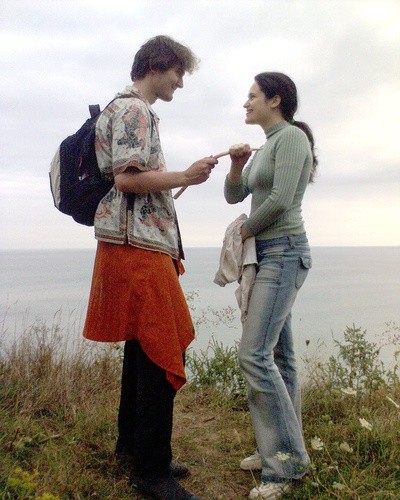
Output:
[248,478,296,500]
[240,448,261,471]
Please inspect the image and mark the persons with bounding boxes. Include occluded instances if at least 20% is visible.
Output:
[223,72,314,500]
[79,35,218,500]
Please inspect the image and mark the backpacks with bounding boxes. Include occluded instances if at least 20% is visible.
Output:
[49,95,155,225]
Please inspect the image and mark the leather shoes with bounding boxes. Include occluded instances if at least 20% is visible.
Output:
[116,455,190,478]
[130,471,199,500]
